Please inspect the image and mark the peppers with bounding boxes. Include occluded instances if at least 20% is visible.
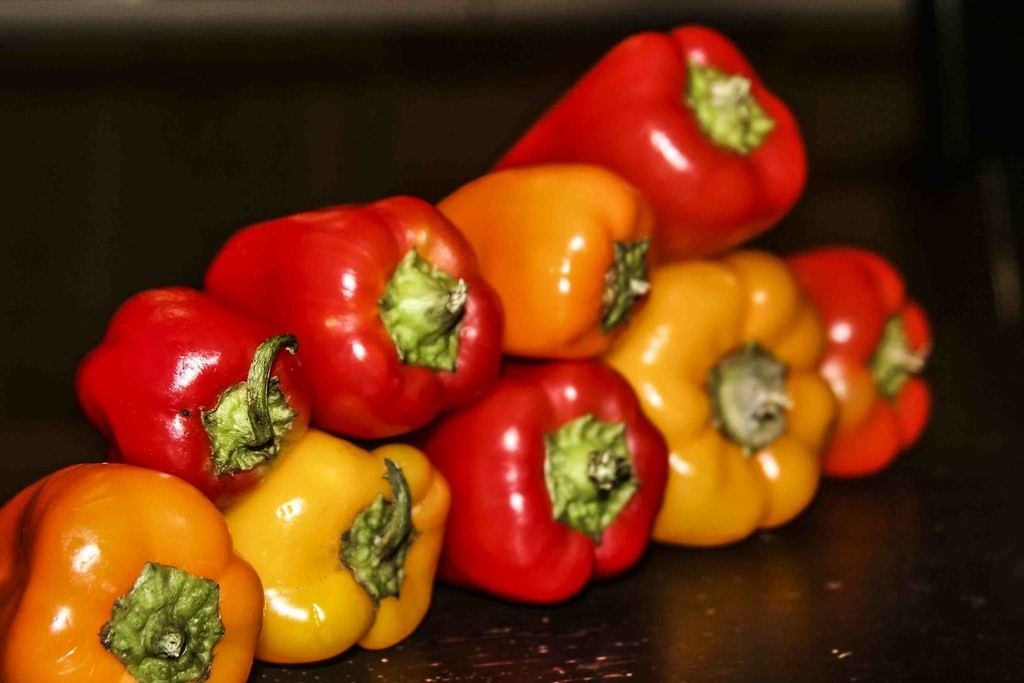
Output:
[0,25,932,683]
